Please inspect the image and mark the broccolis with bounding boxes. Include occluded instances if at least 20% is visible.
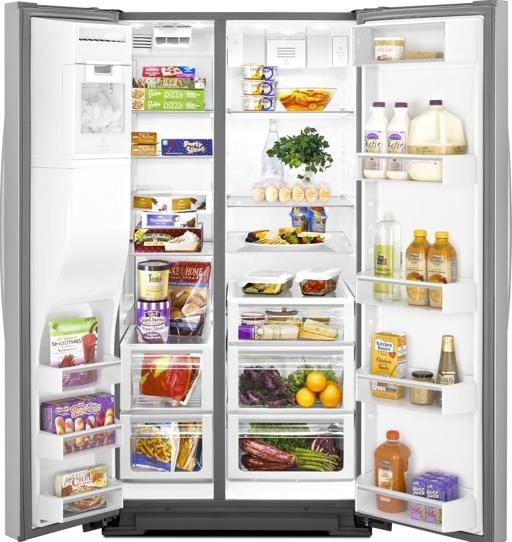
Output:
[1,0,512,542]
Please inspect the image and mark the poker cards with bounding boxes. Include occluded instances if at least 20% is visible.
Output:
[292,446,339,471]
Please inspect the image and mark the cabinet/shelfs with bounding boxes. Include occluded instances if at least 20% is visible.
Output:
[374,428,411,515]
[410,367,435,405]
[260,117,285,189]
[434,335,459,407]
[362,101,389,180]
[405,229,432,307]
[373,212,403,304]
[425,230,458,309]
[386,102,413,181]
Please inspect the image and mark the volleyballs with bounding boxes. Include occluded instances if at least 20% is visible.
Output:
[239,438,298,471]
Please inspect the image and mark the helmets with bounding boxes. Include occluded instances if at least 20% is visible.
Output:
[244,230,335,249]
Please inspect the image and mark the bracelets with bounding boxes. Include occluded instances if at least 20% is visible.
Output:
[272,87,338,113]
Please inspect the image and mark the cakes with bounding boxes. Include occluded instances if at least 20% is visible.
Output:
[411,370,435,405]
